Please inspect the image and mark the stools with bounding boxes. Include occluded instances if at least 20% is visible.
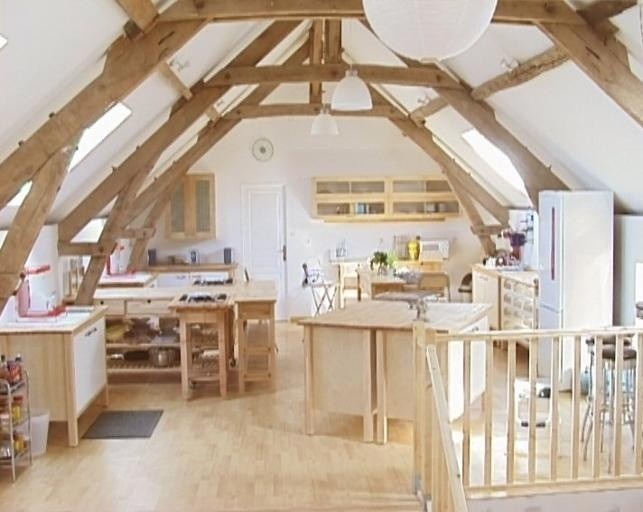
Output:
[580,326,636,474]
[504,377,562,459]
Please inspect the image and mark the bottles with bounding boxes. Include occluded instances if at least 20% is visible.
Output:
[147,248,155,266]
[0,353,27,459]
[223,248,230,264]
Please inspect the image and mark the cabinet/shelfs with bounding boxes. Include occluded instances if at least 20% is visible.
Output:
[471,264,501,347]
[501,275,539,350]
[0,315,109,447]
[93,287,234,373]
[165,173,216,239]
[312,175,463,221]
[0,368,33,482]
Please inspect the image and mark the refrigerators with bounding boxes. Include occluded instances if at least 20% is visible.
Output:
[536,190,616,392]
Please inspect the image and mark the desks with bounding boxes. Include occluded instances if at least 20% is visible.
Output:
[168,283,232,400]
[234,280,278,395]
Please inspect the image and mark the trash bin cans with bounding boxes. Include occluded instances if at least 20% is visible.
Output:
[457,274,471,303]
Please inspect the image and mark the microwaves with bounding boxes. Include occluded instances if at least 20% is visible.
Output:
[418,239,449,261]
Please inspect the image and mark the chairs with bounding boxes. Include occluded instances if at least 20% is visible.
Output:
[302,258,451,317]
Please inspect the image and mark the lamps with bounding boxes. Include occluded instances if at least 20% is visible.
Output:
[310,81,338,136]
[363,0,498,64]
[331,18,373,112]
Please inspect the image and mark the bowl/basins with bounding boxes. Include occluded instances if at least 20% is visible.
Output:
[148,347,179,367]
[105,324,125,341]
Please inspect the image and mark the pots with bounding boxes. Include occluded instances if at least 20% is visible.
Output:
[110,350,150,361]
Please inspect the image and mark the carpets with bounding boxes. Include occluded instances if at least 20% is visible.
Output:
[81,410,163,438]
